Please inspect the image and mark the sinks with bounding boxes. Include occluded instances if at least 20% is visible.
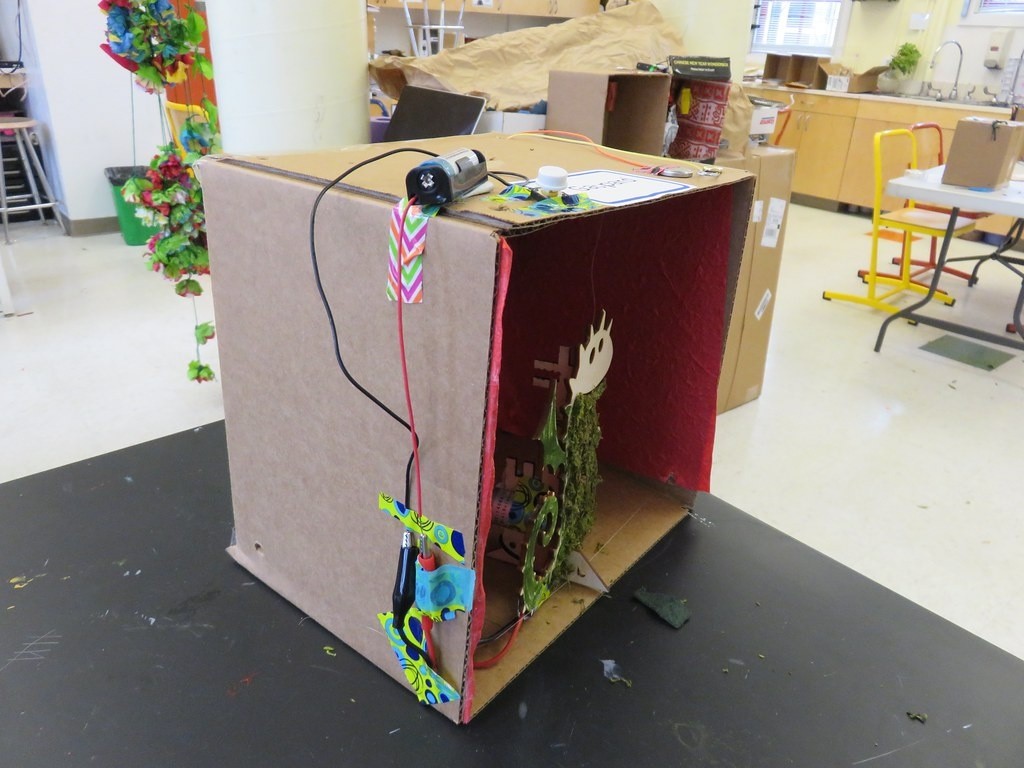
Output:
[911,97,964,103]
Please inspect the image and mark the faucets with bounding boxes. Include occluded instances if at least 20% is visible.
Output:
[929,36,964,104]
[1004,49,1024,106]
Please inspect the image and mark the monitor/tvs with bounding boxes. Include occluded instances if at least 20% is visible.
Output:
[747,0,851,57]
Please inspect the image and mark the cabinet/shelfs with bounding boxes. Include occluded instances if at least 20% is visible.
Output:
[847,100,1012,227]
[740,87,859,212]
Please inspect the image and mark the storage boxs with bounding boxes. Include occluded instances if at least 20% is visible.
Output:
[187,128,757,721]
[761,51,791,85]
[714,143,797,412]
[818,62,890,92]
[698,146,761,414]
[785,54,831,87]
[474,109,549,136]
[544,66,673,160]
[944,114,1024,191]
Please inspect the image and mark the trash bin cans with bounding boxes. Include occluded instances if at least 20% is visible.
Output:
[104,165,161,247]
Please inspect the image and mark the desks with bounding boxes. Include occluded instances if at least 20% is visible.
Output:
[0,73,25,91]
[873,160,1024,353]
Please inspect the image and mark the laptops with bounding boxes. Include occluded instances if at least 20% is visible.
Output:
[382,84,487,142]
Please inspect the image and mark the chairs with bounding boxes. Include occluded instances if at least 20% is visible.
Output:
[823,128,955,328]
[856,121,979,296]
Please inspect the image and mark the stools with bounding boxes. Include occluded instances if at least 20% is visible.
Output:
[0,115,68,246]
